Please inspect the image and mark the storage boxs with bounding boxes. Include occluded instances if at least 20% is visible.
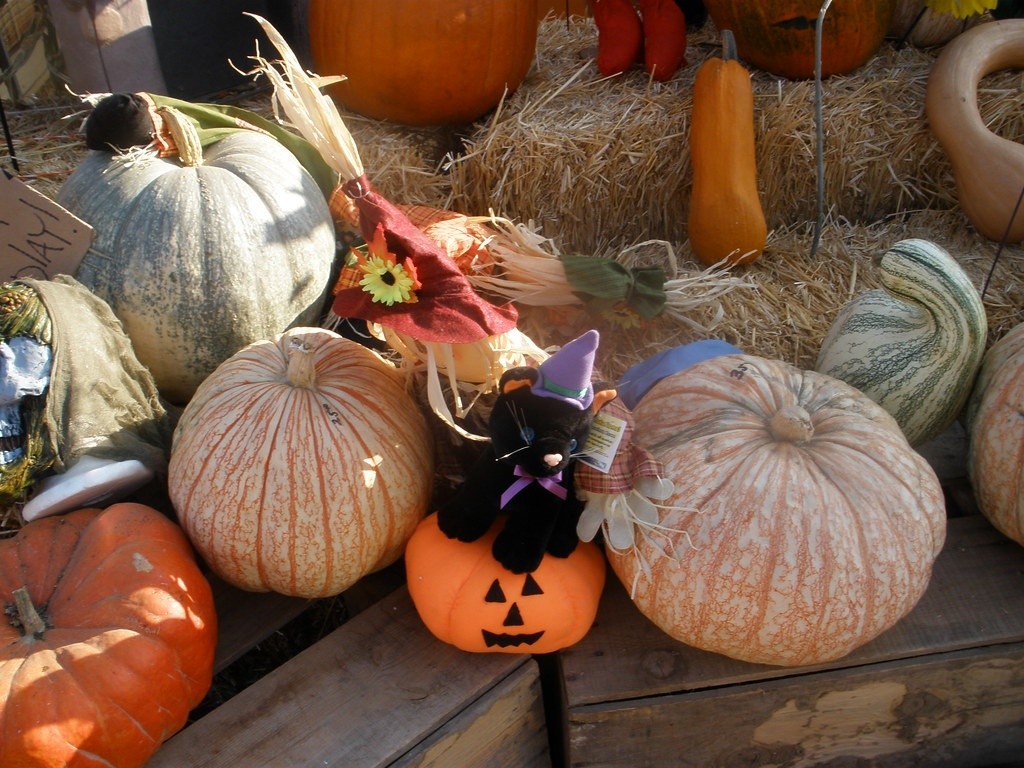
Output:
[554,430,1024,768]
[141,551,553,768]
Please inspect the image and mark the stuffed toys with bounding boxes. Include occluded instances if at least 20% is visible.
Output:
[439,326,599,574]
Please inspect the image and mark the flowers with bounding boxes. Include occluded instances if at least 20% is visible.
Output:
[358,253,413,306]
[922,0,998,20]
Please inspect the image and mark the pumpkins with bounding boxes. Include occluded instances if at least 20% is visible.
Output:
[0,0,1024,768]
